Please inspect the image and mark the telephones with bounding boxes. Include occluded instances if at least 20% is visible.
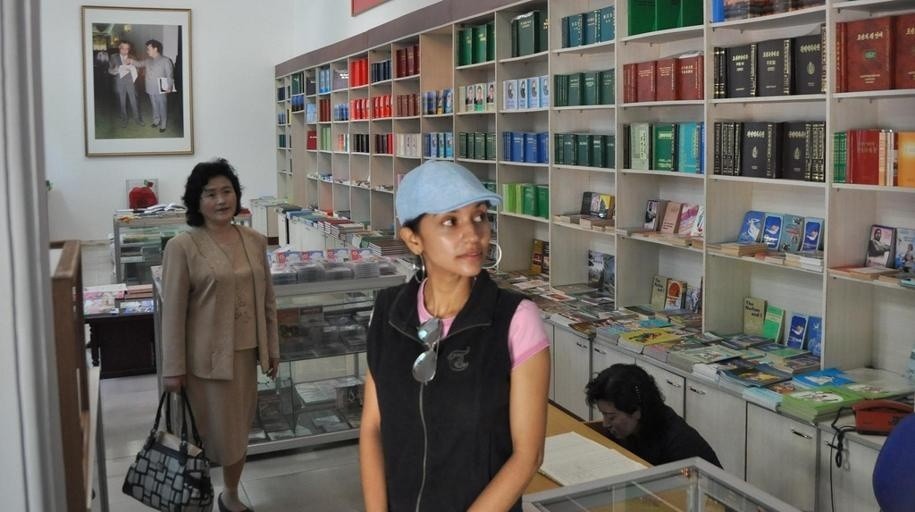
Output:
[852,400,912,435]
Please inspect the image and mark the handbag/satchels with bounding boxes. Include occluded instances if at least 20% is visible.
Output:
[121,430,214,512]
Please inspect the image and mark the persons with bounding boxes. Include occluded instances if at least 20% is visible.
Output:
[159,157,281,511]
[124,40,182,132]
[357,159,551,512]
[872,410,915,511]
[584,362,725,473]
[106,39,145,129]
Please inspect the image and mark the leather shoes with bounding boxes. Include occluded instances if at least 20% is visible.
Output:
[217,492,254,512]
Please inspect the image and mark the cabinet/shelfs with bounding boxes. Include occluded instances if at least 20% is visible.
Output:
[252,200,278,245]
[152,267,406,457]
[50,241,107,512]
[113,214,251,284]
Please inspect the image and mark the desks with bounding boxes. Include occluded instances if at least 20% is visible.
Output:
[85,315,160,381]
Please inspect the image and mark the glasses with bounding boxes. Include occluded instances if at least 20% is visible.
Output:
[412,317,443,384]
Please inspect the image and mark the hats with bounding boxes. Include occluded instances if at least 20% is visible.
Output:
[396,159,503,227]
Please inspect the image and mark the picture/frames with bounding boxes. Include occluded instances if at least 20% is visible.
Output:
[81,7,193,156]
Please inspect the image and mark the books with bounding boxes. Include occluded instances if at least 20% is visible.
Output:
[624,0,703,102]
[503,75,548,162]
[714,2,826,98]
[644,296,914,422]
[278,68,348,151]
[490,239,614,325]
[84,200,275,446]
[536,429,649,490]
[423,87,453,159]
[554,68,615,169]
[459,132,496,193]
[828,225,915,290]
[157,76,177,93]
[268,247,395,442]
[561,5,614,48]
[715,120,825,182]
[833,129,915,187]
[835,0,915,93]
[706,210,823,273]
[275,202,370,243]
[513,9,548,58]
[568,278,702,352]
[352,221,421,272]
[616,122,705,249]
[396,45,419,157]
[350,59,391,155]
[502,181,615,233]
[459,24,495,112]
